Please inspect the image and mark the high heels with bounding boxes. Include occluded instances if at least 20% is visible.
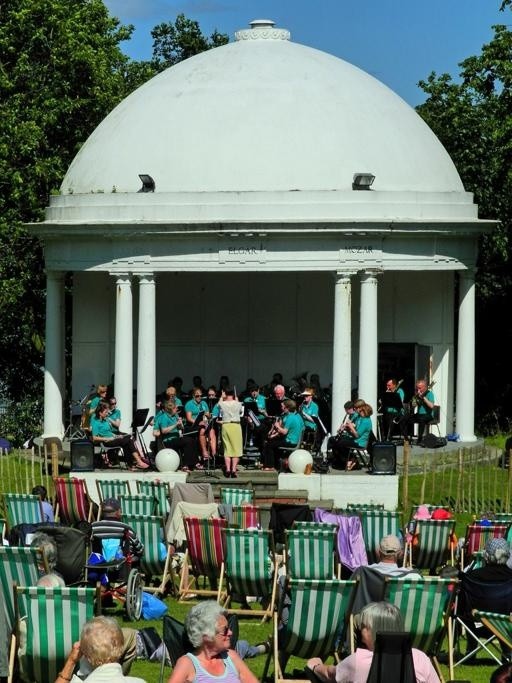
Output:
[225,470,237,478]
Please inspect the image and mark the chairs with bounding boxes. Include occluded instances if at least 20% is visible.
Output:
[382,576,459,683]
[84,403,444,471]
[459,609,510,669]
[2,478,509,604]
[217,527,281,623]
[7,581,102,683]
[271,574,360,683]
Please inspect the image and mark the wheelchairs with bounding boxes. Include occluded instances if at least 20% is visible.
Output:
[83,527,147,620]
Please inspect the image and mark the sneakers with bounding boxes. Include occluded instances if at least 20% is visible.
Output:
[255,640,272,653]
[136,463,149,468]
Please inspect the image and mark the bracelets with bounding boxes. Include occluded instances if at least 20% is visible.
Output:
[312,663,320,672]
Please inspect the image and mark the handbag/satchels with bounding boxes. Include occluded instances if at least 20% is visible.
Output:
[141,626,162,651]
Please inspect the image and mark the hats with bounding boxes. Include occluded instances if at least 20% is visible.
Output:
[250,383,259,391]
[413,505,452,520]
[486,537,510,556]
[102,498,121,513]
[300,389,313,395]
[380,535,400,556]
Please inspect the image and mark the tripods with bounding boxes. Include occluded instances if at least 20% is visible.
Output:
[68,407,91,441]
[193,429,220,480]
[136,426,158,472]
[62,402,82,442]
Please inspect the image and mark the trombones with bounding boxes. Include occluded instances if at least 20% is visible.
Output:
[392,380,437,425]
[377,379,405,412]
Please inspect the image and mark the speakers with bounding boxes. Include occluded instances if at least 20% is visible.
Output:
[369,440,396,475]
[70,443,95,472]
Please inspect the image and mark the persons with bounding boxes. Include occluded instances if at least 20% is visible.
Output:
[17,485,147,682]
[86,385,150,468]
[377,378,434,446]
[153,373,331,478]
[327,400,373,471]
[306,601,442,683]
[456,537,512,664]
[365,534,422,580]
[431,508,458,554]
[400,506,432,536]
[168,599,260,683]
